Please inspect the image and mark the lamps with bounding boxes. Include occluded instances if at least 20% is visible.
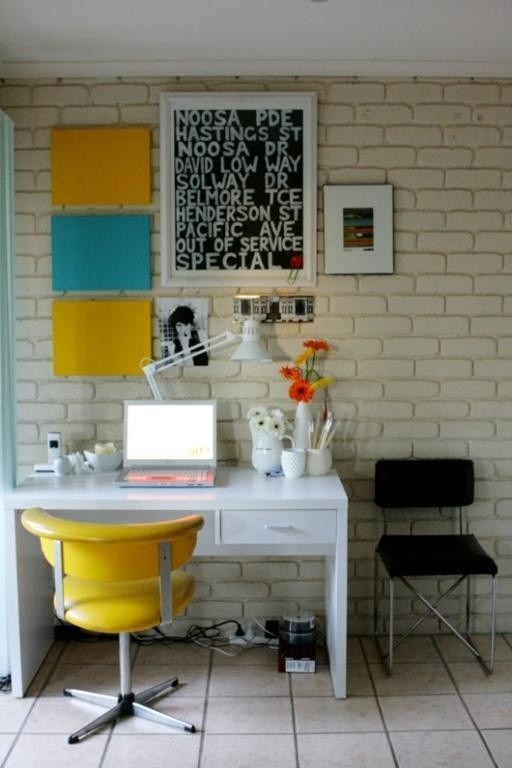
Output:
[142,317,275,400]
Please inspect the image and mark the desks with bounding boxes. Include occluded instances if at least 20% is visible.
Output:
[4,466,346,699]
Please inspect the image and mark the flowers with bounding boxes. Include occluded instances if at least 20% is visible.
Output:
[246,409,287,440]
[281,337,336,403]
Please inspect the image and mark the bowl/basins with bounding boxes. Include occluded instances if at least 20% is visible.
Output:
[83,449,123,472]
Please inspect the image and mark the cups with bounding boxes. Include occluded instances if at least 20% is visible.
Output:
[281,447,306,478]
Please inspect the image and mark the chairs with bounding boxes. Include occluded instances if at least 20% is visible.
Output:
[372,459,498,676]
[21,506,205,745]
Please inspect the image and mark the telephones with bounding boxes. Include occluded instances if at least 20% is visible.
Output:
[33,432,62,471]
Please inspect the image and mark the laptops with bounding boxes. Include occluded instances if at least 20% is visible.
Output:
[113,401,218,489]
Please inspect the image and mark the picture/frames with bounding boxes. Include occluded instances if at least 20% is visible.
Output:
[161,90,319,289]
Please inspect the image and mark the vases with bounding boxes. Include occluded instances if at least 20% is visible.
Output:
[290,404,314,450]
[254,428,283,480]
